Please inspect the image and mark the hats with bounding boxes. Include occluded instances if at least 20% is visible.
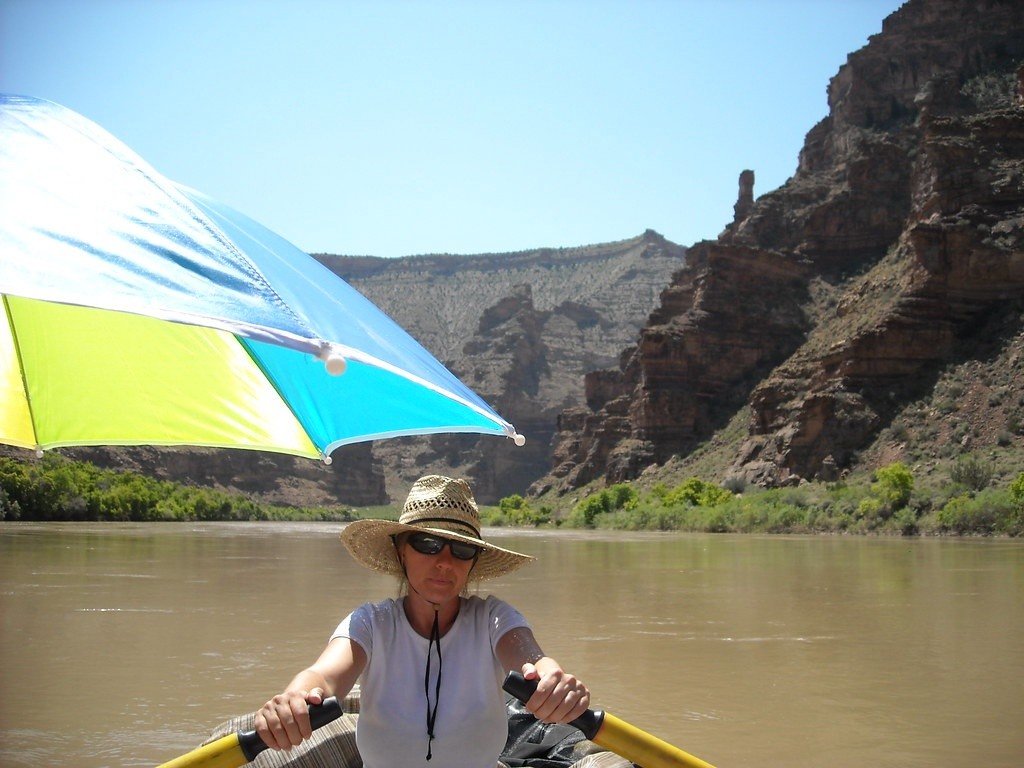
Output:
[340,475,537,583]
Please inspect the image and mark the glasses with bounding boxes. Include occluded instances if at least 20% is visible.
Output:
[400,532,479,560]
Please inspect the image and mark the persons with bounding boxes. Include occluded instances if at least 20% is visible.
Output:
[255,475,590,768]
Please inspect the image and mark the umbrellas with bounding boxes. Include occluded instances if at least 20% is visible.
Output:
[1,93,526,465]
